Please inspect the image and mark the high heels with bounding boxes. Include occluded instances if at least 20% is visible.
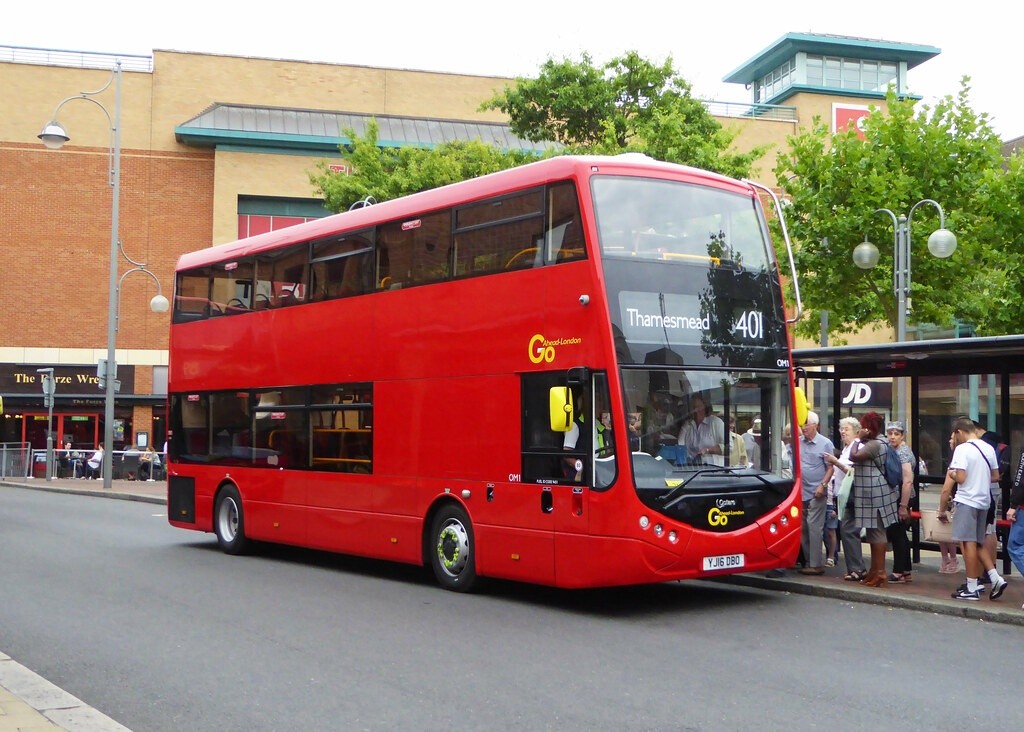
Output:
[859,571,882,586]
[866,571,888,587]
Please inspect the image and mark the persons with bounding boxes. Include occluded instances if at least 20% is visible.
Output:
[936,416,1024,609]
[59,441,82,476]
[79,442,104,480]
[784,410,916,587]
[645,394,788,469]
[563,391,611,482]
[122,438,161,480]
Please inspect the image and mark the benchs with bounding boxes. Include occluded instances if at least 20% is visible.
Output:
[906,512,922,532]
[996,520,1012,542]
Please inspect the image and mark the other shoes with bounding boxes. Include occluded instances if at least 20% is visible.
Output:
[798,567,825,575]
[844,569,867,580]
[905,573,913,582]
[888,573,906,583]
[957,580,985,592]
[824,558,835,567]
[939,559,958,573]
[766,569,783,578]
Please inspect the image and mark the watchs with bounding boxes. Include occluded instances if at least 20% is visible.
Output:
[854,438,861,443]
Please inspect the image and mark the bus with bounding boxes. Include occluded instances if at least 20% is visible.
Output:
[168,154,812,594]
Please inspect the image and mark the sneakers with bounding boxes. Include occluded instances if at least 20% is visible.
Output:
[990,577,1008,600]
[951,590,979,600]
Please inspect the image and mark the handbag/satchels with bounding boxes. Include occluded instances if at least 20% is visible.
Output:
[986,492,997,524]
[836,469,854,521]
[921,510,958,543]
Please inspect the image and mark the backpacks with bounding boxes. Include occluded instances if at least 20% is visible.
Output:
[870,439,903,488]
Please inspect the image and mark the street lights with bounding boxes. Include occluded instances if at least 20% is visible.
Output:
[36,368,54,481]
[853,199,957,442]
[38,60,169,488]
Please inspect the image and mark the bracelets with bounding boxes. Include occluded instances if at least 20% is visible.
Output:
[706,448,709,453]
[821,482,828,487]
[899,503,908,508]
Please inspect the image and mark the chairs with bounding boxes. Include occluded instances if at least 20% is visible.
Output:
[58,455,165,482]
[545,431,564,473]
[189,425,373,474]
[203,277,391,318]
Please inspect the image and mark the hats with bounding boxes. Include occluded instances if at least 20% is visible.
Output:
[748,419,772,436]
[886,420,904,432]
